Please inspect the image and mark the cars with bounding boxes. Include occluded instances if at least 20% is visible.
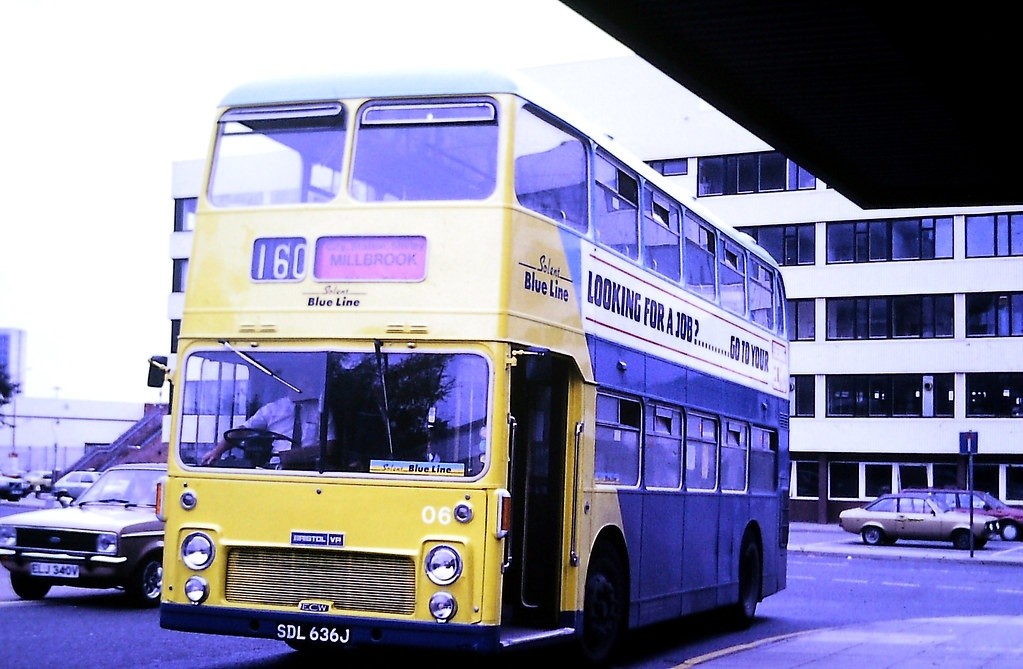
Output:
[1,470,52,502]
[839,493,1001,550]
[0,464,194,610]
[902,489,1023,542]
[52,471,130,501]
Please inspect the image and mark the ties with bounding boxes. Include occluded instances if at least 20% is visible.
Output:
[291,406,302,448]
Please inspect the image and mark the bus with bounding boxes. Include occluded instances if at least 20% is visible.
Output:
[147,93,794,664]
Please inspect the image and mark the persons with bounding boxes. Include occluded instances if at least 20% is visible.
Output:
[199,366,337,467]
